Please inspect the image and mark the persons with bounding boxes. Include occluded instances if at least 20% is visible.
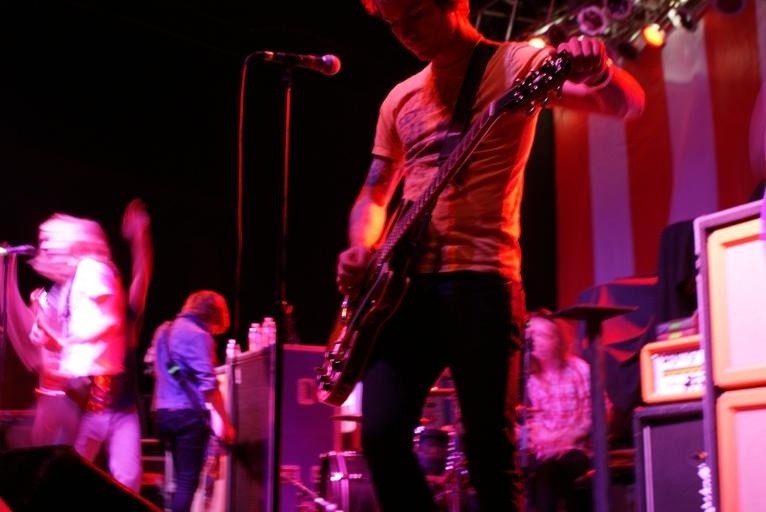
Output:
[140,288,239,511]
[332,0,651,510]
[409,425,473,512]
[89,196,155,500]
[0,210,130,467]
[516,305,618,511]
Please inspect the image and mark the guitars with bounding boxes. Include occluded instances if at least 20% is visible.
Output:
[315,49,569,407]
[205,391,236,446]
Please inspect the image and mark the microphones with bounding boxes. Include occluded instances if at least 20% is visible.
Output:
[256,48,342,79]
[0,245,36,256]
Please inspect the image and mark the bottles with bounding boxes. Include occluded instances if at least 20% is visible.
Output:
[248,323,262,351]
[262,317,277,348]
[226,339,237,362]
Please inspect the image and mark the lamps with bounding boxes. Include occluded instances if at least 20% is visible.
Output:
[617,1,711,59]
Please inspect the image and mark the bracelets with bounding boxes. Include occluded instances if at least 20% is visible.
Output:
[583,68,612,96]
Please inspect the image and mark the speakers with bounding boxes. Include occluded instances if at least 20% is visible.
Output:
[693,198,766,512]
[632,400,711,512]
[226,342,341,512]
